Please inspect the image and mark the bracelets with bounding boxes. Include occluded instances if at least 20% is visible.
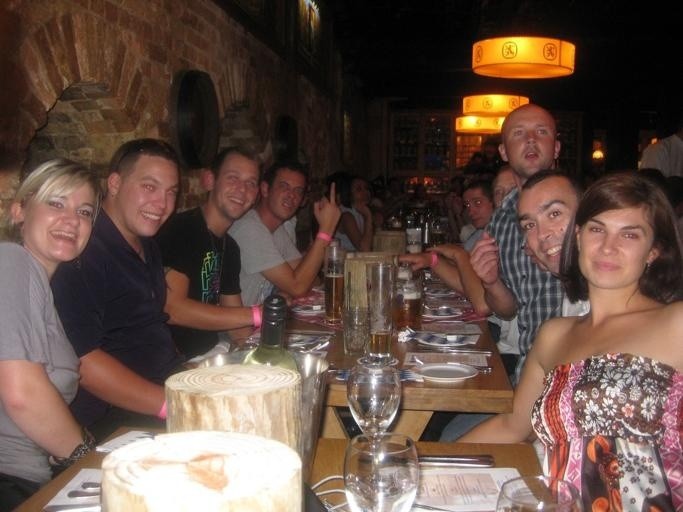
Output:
[252,304,261,327]
[49,428,98,476]
[159,401,167,419]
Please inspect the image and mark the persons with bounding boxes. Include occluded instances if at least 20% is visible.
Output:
[457,171,683,509]
[228,161,342,306]
[438,174,591,442]
[312,171,385,273]
[638,129,682,172]
[49,138,182,435]
[397,105,565,385]
[1,157,104,509]
[158,139,264,356]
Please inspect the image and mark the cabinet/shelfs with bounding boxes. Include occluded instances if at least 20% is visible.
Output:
[388,109,456,195]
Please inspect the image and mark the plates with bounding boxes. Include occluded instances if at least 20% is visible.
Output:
[239,207,494,386]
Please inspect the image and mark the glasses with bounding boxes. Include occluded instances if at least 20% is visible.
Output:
[118,138,179,170]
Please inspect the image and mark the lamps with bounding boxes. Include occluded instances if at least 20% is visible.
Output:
[472,36,576,79]
[456,116,506,134]
[463,94,530,118]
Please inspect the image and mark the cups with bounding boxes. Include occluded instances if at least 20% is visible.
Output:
[344,431,421,511]
[495,474,577,511]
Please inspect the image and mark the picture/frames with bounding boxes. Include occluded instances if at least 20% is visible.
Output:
[340,108,352,167]
[217,0,288,61]
[288,0,336,105]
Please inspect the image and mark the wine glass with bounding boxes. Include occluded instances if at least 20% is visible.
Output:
[345,360,405,460]
